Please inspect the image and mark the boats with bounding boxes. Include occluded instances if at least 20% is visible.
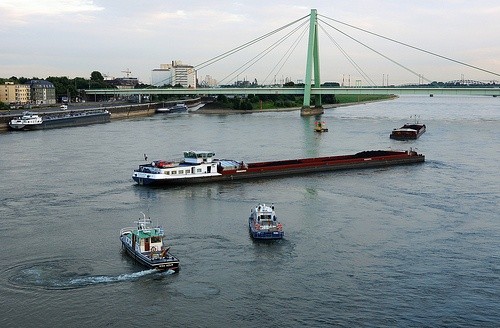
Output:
[248,201,284,240]
[313,118,328,132]
[390,122,426,141]
[154,104,188,114]
[119,210,181,271]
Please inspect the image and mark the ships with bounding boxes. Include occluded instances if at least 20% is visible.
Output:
[7,108,113,129]
[132,149,426,188]
